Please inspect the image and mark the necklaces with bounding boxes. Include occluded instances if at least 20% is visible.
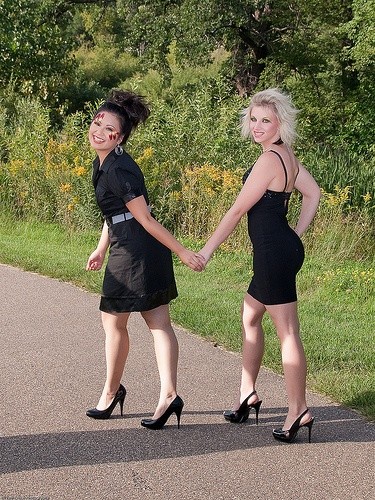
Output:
[262,136,280,152]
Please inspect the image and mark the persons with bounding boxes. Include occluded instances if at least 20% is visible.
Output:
[85,90,205,430]
[196,86,321,444]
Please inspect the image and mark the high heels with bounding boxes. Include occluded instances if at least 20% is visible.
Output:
[222,390,263,426]
[139,394,185,431]
[85,384,128,420]
[272,407,316,444]
